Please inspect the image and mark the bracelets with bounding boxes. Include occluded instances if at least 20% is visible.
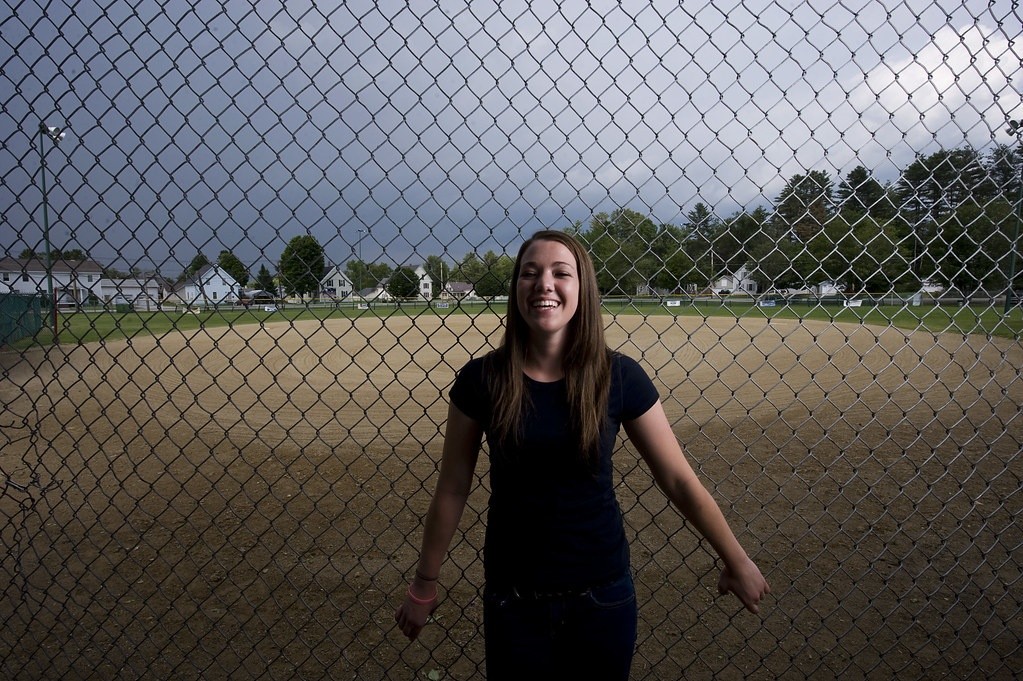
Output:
[407,587,439,605]
[415,569,439,581]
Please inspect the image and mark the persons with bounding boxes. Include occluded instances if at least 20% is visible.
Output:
[396,230,770,680]
